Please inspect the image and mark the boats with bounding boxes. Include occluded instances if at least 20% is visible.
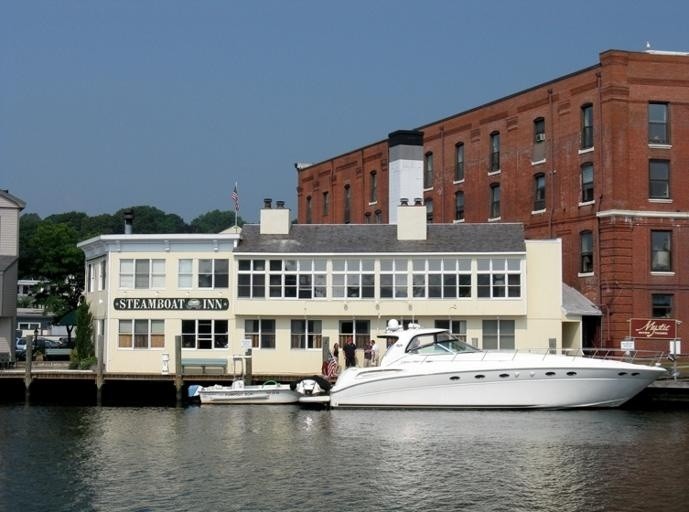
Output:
[330,319,669,410]
[197,380,303,405]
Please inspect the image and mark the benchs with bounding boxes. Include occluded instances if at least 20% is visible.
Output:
[181,358,228,375]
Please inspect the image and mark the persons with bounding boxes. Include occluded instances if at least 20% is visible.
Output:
[333,342,340,375]
[370,339,377,367]
[364,340,373,367]
[343,336,357,368]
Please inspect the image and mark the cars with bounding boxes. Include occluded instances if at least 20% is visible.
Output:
[16,336,74,350]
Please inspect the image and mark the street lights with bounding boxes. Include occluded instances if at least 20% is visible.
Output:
[34,328,38,352]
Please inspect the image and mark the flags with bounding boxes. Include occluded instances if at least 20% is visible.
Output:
[327,352,338,380]
[232,185,241,212]
